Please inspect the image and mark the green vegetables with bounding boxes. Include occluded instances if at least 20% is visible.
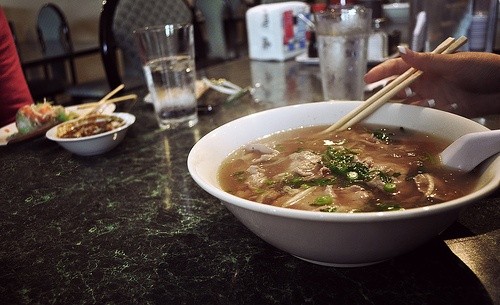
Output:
[16,102,82,136]
[229,123,440,212]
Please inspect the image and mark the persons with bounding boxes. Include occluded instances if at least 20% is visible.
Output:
[0,4,33,144]
[364,47,500,132]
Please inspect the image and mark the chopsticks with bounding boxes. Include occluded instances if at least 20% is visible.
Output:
[77,84,137,116]
[312,34,468,140]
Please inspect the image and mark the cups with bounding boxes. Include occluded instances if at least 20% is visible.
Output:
[312,4,373,101]
[133,23,199,130]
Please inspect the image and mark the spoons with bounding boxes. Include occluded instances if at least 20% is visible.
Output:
[439,130,500,173]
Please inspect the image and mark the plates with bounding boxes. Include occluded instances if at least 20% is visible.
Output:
[0,101,116,147]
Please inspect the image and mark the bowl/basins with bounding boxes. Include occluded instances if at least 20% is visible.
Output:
[187,100,500,270]
[382,2,410,25]
[45,111,136,156]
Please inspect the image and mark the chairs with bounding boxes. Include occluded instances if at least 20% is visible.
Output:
[8,0,249,106]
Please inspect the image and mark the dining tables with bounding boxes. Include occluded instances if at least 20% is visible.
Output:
[0,56,500,305]
